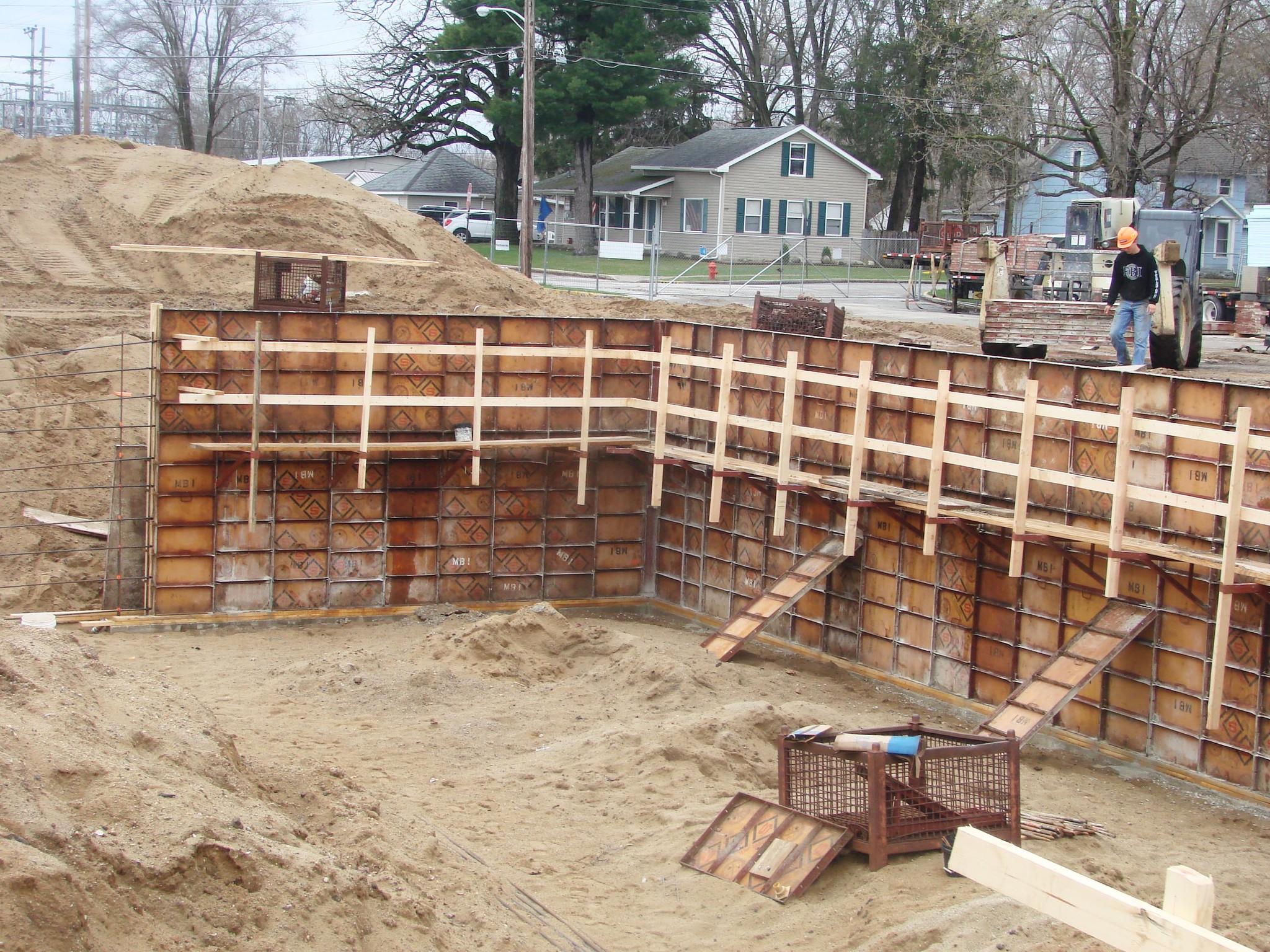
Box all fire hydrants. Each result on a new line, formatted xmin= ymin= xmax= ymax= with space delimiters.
xmin=707 ymin=262 xmax=718 ymax=280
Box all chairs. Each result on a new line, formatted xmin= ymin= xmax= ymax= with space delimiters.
xmin=544 ymin=231 xmax=555 ymax=244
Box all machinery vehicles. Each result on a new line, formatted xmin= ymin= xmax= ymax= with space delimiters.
xmin=976 ymin=192 xmax=1205 ymax=370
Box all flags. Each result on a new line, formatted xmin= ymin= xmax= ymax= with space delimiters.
xmin=591 ymin=202 xmax=598 ymax=216
xmin=536 ymin=195 xmax=553 ymax=234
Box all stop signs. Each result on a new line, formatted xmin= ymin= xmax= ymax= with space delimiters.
xmin=938 ymin=224 xmax=964 ymax=247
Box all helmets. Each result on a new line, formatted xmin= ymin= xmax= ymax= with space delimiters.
xmin=1117 ymin=227 xmax=1138 ymax=248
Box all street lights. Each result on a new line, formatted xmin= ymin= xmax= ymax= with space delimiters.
xmin=475 ymin=7 xmax=534 ymax=276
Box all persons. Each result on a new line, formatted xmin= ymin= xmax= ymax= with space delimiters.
xmin=1104 ymin=226 xmax=1160 ymax=369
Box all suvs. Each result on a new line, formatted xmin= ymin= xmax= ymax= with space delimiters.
xmin=444 ymin=209 xmax=544 ymax=244
xmin=416 ymin=205 xmax=460 ymax=224
